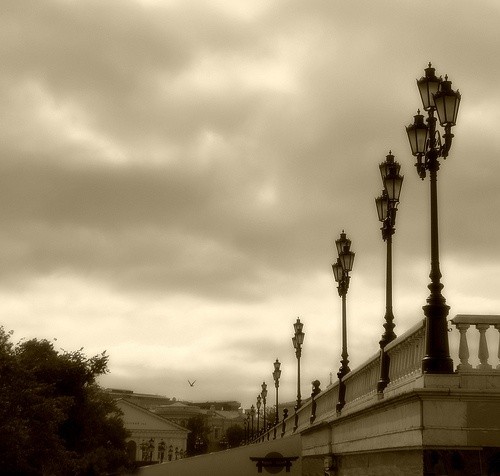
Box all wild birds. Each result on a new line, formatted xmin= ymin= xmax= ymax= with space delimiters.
xmin=187 ymin=377 xmax=199 ymax=389
